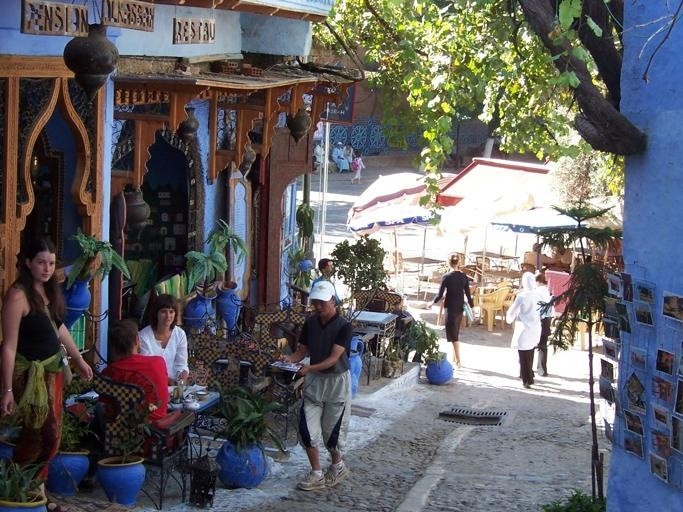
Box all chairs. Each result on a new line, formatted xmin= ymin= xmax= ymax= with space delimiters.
xmin=457 ymin=253 xmax=476 ymax=275
xmin=496 ymin=280 xmax=508 ymax=289
xmin=478 ymin=287 xmax=512 ymax=332
xmin=92 ymin=369 xmax=196 ymax=510
xmin=269 ymin=322 xmax=299 ymax=353
xmin=476 ymin=257 xmax=496 ymax=284
xmin=577 ymin=253 xmax=624 ymax=273
xmin=368 ymin=299 xmax=386 ymax=313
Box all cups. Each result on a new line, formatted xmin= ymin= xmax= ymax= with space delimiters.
xmin=172 ymin=388 xmax=182 ymax=404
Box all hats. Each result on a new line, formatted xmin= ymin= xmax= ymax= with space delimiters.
xmin=309 ymin=281 xmax=336 ymax=301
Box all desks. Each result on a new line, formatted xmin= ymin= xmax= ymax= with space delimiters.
xmin=400 ymin=257 xmax=446 ymax=301
xmin=489 ymin=270 xmax=522 ymax=289
xmin=470 ymin=251 xmax=520 ymax=270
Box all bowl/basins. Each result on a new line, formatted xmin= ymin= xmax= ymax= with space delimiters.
xmin=196 ymin=392 xmax=209 ymax=400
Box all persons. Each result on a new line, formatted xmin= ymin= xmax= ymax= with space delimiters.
xmin=314 ymin=141 xmax=333 ymax=174
xmin=532 ymin=275 xmax=554 ymax=376
xmin=505 ymin=271 xmax=542 ymax=389
xmin=332 ymin=142 xmax=349 ymax=173
xmin=428 ymin=253 xmax=475 ymax=368
xmin=351 ymin=152 xmax=365 ymax=184
xmin=392 ymin=299 xmax=430 ymax=363
xmin=277 ymin=281 xmax=354 ymax=491
xmin=311 ymin=258 xmax=341 ymax=307
xmin=2 ymin=235 xmax=93 ymax=511
xmin=343 ymin=142 xmax=356 ymax=172
xmin=100 ymin=318 xmax=168 ymax=423
xmin=525 ymin=242 xmax=555 ymax=271
xmin=138 ymin=294 xmax=189 ymax=386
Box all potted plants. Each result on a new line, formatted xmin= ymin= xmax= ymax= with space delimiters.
xmin=204 ymin=219 xmax=248 ymax=340
xmin=57 ymin=227 xmax=131 ymax=331
xmin=0 ymin=419 xmax=23 ymax=460
xmin=382 ymin=340 xmax=405 ymax=378
xmin=407 ymin=320 xmax=454 ymax=385
xmin=214 ymin=380 xmax=283 ymax=489
xmin=97 ymin=400 xmax=163 ymax=509
xmin=183 ymin=251 xmax=228 ymax=338
xmin=0 ymin=456 xmax=47 ymax=512
xmin=43 ymin=409 xmax=89 ymax=496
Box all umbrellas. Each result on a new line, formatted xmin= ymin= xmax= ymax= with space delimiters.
xmin=348 ymin=173 xmax=428 ymax=225
xmin=350 ymin=204 xmax=441 ymax=277
xmin=491 ymin=201 xmax=623 ymax=267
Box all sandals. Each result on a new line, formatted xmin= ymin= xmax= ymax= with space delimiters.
xmin=46 ymin=497 xmax=68 ymax=512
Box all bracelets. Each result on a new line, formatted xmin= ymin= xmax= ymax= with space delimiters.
xmin=3 ymin=388 xmax=13 ymax=392
xmin=72 ymin=354 xmax=83 ymax=361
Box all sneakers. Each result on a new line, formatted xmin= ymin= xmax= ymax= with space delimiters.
xmin=325 ymin=462 xmax=349 ymax=487
xmin=297 ymin=471 xmax=325 ymax=489
